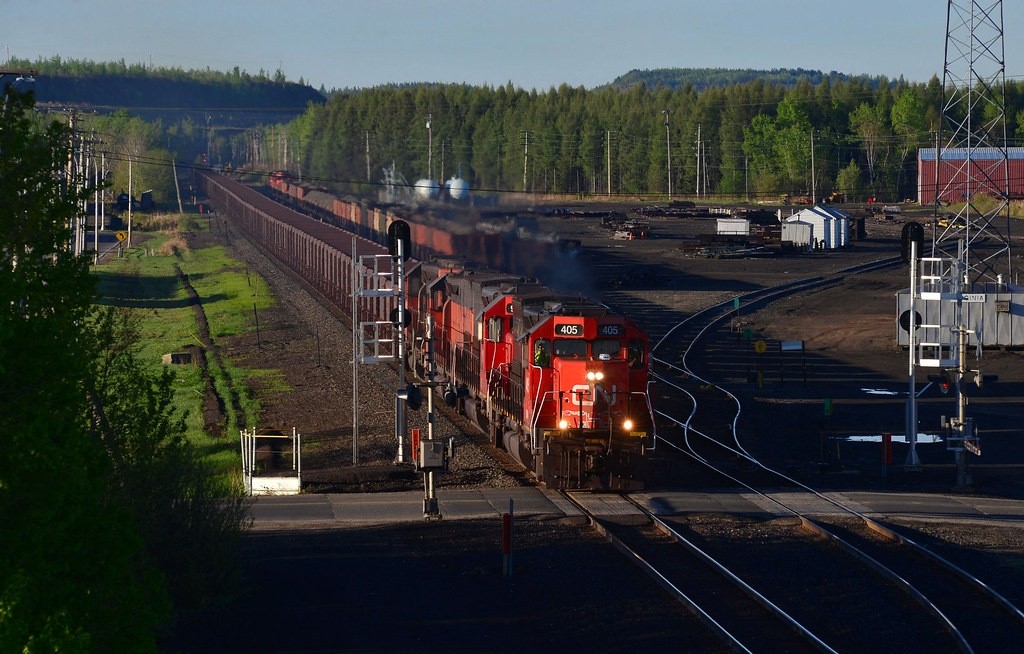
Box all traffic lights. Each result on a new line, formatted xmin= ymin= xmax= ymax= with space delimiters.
xmin=443 ymin=391 xmax=457 ymax=408
xmin=407 ymin=388 xmax=422 ymax=411
xmin=973 ymin=369 xmax=1000 ymax=389
xmin=927 ymin=370 xmax=951 ymax=393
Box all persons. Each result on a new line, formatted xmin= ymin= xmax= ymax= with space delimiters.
xmin=535 ymin=344 xmax=549 ymax=365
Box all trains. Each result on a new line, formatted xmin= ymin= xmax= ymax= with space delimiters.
xmin=190 ymin=164 xmax=657 ymax=493
xmin=269 ymin=170 xmax=583 ymax=285
